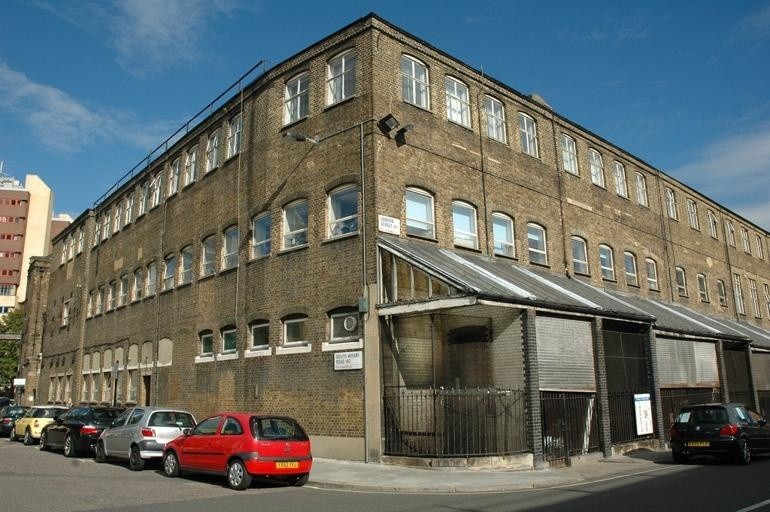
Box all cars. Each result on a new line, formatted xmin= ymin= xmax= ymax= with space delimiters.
xmin=670 ymin=402 xmax=770 ymax=465
